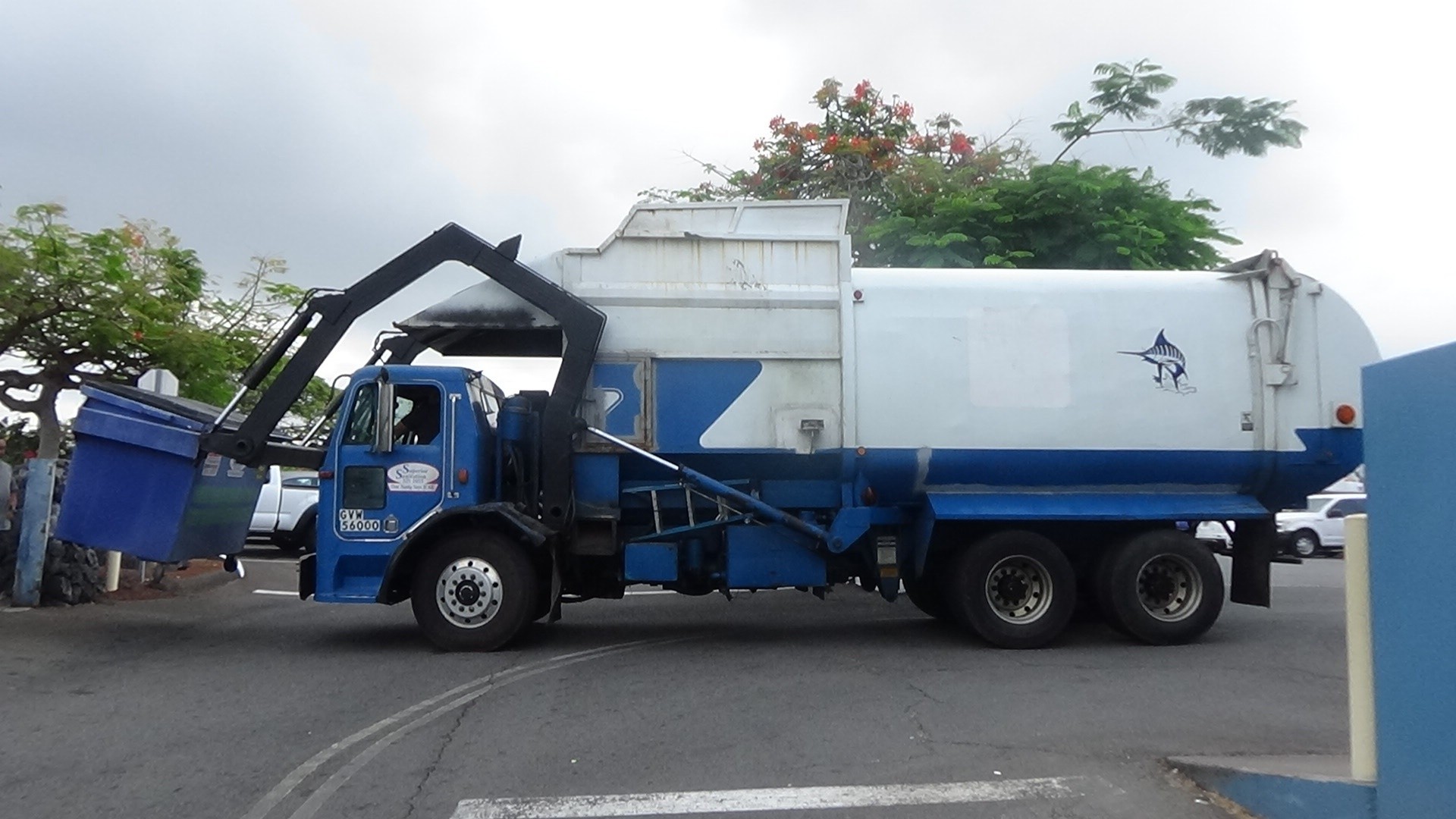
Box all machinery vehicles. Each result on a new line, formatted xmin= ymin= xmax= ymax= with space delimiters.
xmin=48 ymin=192 xmax=1387 ymax=650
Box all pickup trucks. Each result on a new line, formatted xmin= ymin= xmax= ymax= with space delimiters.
xmin=244 ymin=463 xmax=323 ymax=551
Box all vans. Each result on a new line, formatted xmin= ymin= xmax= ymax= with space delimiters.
xmin=1192 ymin=491 xmax=1369 ymax=558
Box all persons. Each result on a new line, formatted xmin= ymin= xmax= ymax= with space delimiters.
xmin=394 ymin=385 xmax=440 ymax=436
